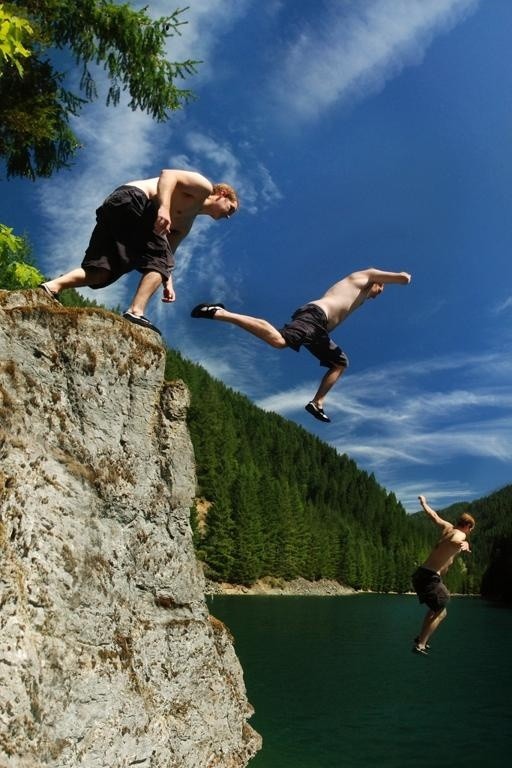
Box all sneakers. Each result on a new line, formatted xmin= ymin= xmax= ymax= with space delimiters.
xmin=411 ymin=637 xmax=433 ymax=659
xmin=188 ymin=302 xmax=226 ymax=320
xmin=305 ymin=400 xmax=333 ymax=424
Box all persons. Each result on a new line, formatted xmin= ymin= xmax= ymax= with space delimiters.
xmin=190 ymin=267 xmax=410 ymax=424
xmin=39 ymin=169 xmax=238 ymax=337
xmin=412 ymin=496 xmax=476 ymax=656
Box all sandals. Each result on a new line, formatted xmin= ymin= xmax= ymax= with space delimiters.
xmin=122 ymin=311 xmax=165 ymax=338
xmin=36 ymin=281 xmax=65 ymax=308
